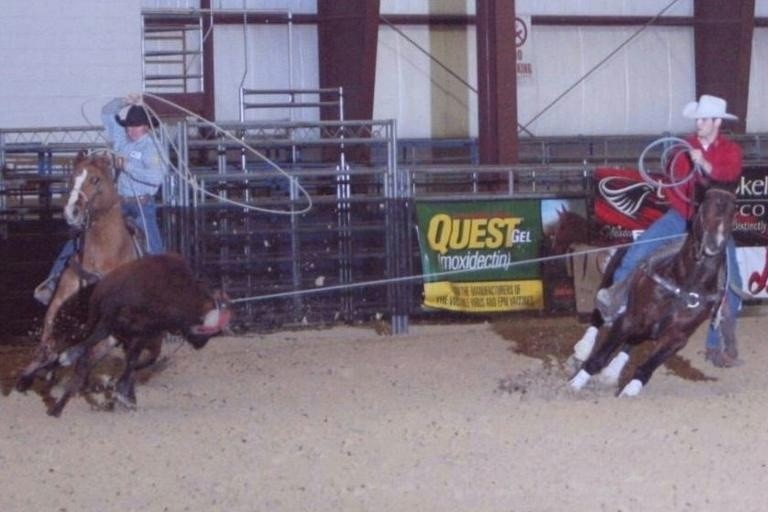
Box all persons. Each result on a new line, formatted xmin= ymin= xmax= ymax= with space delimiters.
xmin=596 ymin=95 xmax=743 ymax=368
xmin=33 ymin=92 xmax=167 ymax=306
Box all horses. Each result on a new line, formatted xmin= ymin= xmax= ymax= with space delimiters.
xmin=38 ymin=148 xmax=144 ymax=391
xmin=566 ymin=176 xmax=740 ymax=400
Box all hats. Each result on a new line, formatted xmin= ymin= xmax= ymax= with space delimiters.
xmin=683 ymin=94 xmax=739 ymax=120
xmin=114 ymin=105 xmax=160 ymax=127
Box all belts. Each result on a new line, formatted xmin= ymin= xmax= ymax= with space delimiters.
xmin=123 ymin=195 xmax=151 ymax=204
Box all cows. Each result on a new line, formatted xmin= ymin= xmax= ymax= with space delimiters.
xmin=15 ymin=251 xmax=239 ymax=413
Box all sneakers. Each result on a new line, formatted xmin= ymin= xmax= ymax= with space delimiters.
xmin=33 ymin=286 xmax=54 ymax=306
xmin=706 ymin=351 xmax=740 ymax=366
